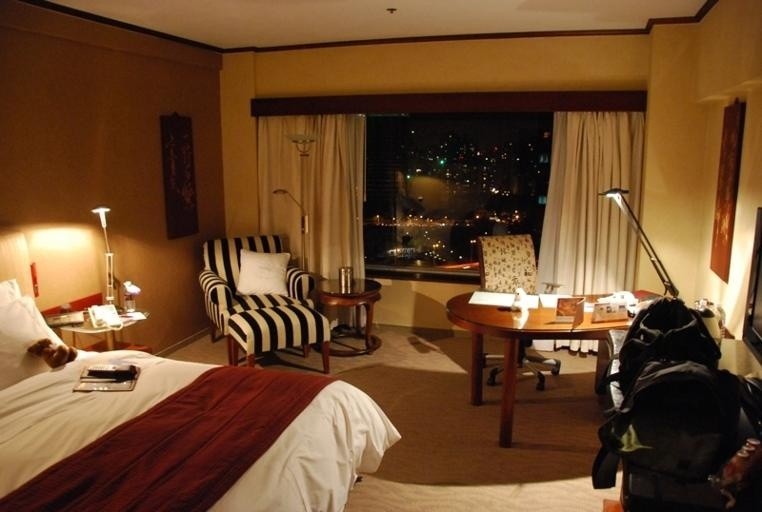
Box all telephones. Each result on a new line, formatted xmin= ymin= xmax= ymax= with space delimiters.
xmin=88 ymin=304 xmax=124 ymax=331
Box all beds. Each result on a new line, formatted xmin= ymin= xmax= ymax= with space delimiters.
xmin=0 ymin=228 xmax=400 ymax=512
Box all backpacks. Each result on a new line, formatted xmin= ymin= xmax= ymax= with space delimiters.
xmin=590 ymin=296 xmax=761 ymax=512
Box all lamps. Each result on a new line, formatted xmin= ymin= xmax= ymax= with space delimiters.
xmin=274 ymin=135 xmax=318 ymax=270
xmin=91 ymin=205 xmax=123 ymax=305
xmin=598 ymin=188 xmax=681 ymax=301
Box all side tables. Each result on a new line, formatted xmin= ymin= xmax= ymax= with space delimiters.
xmin=314 ymin=279 xmax=381 ymax=357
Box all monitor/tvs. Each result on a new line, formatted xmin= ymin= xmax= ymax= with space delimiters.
xmin=749 ymin=249 xmax=762 ymax=359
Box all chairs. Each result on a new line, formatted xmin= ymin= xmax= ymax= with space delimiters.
xmin=478 ymin=234 xmax=561 ymax=390
xmin=199 ymin=235 xmax=314 ymax=363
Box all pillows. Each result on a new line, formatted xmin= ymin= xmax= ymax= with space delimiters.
xmin=0 ymin=297 xmax=66 ymax=387
xmin=0 ymin=279 xmax=21 ymax=305
xmin=235 ymin=248 xmax=291 ymax=296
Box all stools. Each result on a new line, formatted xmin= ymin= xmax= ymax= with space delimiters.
xmin=229 ymin=304 xmax=331 ymax=373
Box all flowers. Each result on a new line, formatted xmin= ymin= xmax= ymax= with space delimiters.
xmin=124 ymin=279 xmax=140 ymax=300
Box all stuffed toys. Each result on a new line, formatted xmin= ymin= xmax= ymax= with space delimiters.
xmin=28 ymin=338 xmax=79 ymax=367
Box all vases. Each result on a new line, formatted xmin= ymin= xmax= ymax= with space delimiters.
xmin=124 ymin=300 xmax=136 ymax=312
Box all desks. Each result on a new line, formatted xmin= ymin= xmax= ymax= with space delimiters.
xmin=605 ymin=330 xmax=762 ymax=512
xmin=56 ymin=309 xmax=152 ymax=353
xmin=447 ymin=291 xmax=662 ymax=448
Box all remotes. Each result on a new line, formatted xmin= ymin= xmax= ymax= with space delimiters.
xmin=87 ymin=363 xmax=132 ymax=375
